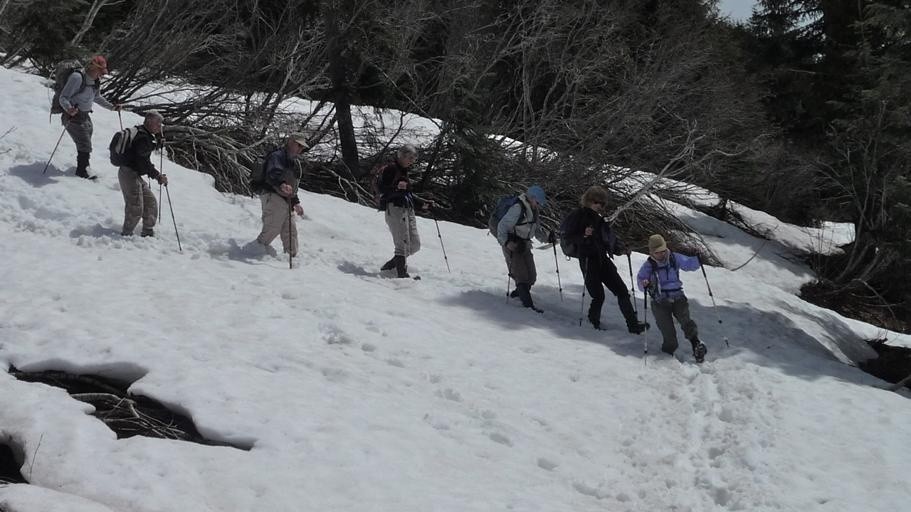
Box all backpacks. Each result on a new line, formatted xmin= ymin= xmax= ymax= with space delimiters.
xmin=107 ymin=123 xmax=147 ymax=167
xmin=560 ymin=211 xmax=584 ymax=258
xmin=249 ymin=162 xmax=268 ymax=195
xmin=372 ymin=163 xmax=389 ymax=198
xmin=488 ymin=191 xmax=527 ymax=239
xmin=49 ymin=58 xmax=87 ymax=114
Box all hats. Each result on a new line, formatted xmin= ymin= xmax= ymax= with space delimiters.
xmin=527 ymin=185 xmax=549 ymax=204
xmin=289 ymin=131 xmax=311 ymax=148
xmin=647 ymin=234 xmax=668 ymax=256
xmin=581 ymin=186 xmax=609 ymax=209
xmin=93 ymin=55 xmax=110 ymax=74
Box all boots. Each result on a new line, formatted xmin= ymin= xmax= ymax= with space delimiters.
xmin=380 ymin=253 xmax=396 ymax=272
xmin=585 ymin=299 xmax=607 ymax=333
xmin=395 ymin=253 xmax=420 ymax=282
xmin=75 ymin=151 xmax=97 ymax=180
xmin=618 ymin=297 xmax=650 ymax=334
xmin=510 ymin=284 xmax=532 ymax=299
xmin=689 ymin=336 xmax=707 ymax=365
xmin=517 ymin=283 xmax=544 ymax=314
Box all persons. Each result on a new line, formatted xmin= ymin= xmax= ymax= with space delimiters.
xmin=379 ymin=144 xmax=422 ymax=281
xmin=636 ymin=234 xmax=708 ymax=362
xmin=495 ymin=186 xmax=549 ymax=314
xmin=560 ymin=185 xmax=651 ymax=335
xmin=251 ymin=130 xmax=310 ymax=257
xmin=118 ymin=109 xmax=168 ymax=236
xmin=58 ymin=55 xmax=122 ymax=179
